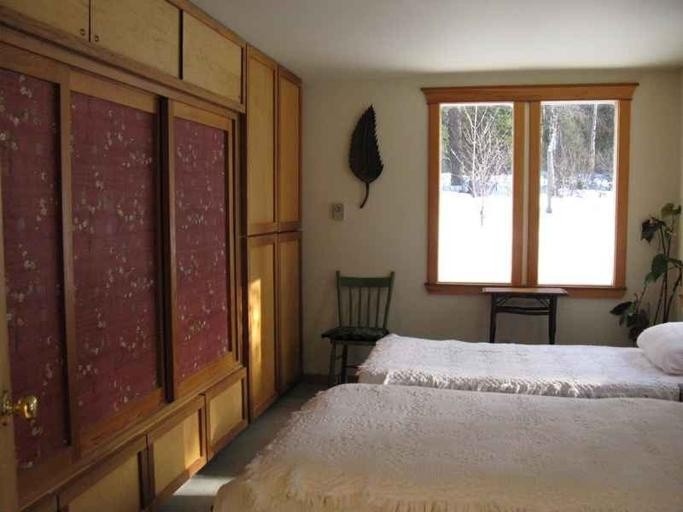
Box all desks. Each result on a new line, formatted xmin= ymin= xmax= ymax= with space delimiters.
xmin=479 ymin=285 xmax=572 ymax=345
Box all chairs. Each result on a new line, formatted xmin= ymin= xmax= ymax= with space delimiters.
xmin=321 ymin=270 xmax=396 ymax=384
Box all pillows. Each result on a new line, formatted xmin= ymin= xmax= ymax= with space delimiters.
xmin=635 ymin=318 xmax=683 ymax=377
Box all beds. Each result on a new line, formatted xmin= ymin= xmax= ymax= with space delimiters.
xmin=354 ymin=332 xmax=682 ymax=401
xmin=207 ymin=381 xmax=682 ymax=512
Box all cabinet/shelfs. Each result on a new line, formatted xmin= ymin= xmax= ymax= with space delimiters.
xmin=245 ymin=50 xmax=276 ymax=421
xmin=149 ymin=367 xmax=248 ymax=502
xmin=181 ymin=11 xmax=242 ymax=105
xmin=275 ymin=60 xmax=302 ymax=402
xmin=3 ymin=0 xmax=179 ymax=79
xmin=0 ymin=43 xmax=243 ymax=511
xmin=56 ymin=429 xmax=151 ymax=511
